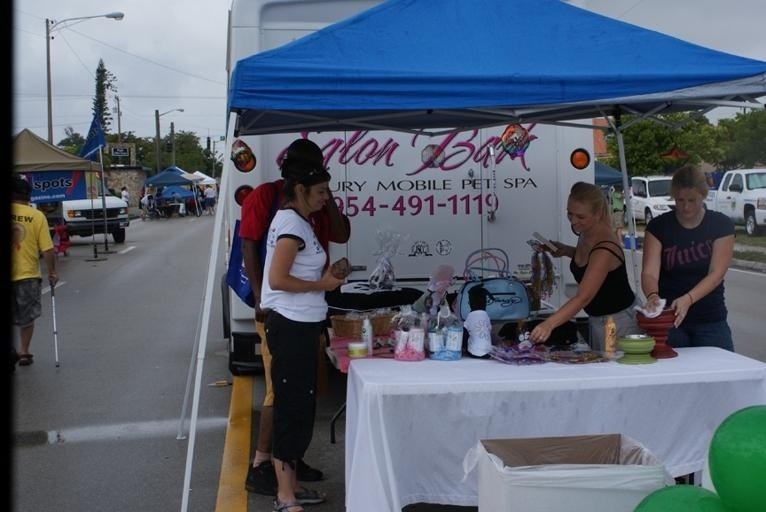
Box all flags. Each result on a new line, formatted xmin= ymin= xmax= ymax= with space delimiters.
xmin=79 ymin=110 xmax=106 ymax=160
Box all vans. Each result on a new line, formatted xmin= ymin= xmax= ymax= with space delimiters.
xmin=59 ymin=178 xmax=130 ymax=243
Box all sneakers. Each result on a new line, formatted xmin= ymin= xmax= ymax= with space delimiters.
xmin=245 ymin=460 xmax=278 ymax=496
xmin=296 ymin=456 xmax=323 ymax=481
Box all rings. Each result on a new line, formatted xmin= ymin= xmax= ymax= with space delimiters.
xmin=538 ymin=334 xmax=544 ymax=343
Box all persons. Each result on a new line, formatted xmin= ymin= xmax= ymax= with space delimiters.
xmin=609 ymin=183 xmax=627 ymax=246
xmin=238 ymin=138 xmax=352 ymax=497
xmin=642 ymin=163 xmax=738 ymax=353
xmin=11 ymin=176 xmax=57 ymax=366
xmin=120 ymin=186 xmax=130 ymax=207
xmin=137 ymin=183 xmax=215 ymax=221
xmin=528 ymin=181 xmax=648 ymax=351
xmin=238 ymin=164 xmax=345 ymax=510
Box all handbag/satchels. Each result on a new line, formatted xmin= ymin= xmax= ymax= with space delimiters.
xmin=225 ymin=182 xmax=279 ymax=308
xmin=454 ymin=248 xmax=531 ymax=322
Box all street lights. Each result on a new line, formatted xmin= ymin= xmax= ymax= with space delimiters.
xmin=42 ymin=8 xmax=128 ymax=150
xmin=150 ymin=108 xmax=186 ymax=172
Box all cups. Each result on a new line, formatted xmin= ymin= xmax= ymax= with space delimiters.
xmin=348 ymin=342 xmax=367 ymax=358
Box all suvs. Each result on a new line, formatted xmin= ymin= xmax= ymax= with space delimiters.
xmin=622 ymin=177 xmax=676 ymax=224
xmin=714 ymin=168 xmax=766 ymax=235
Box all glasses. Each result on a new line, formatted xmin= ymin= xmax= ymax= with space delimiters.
xmin=295 ymin=165 xmax=326 ymax=185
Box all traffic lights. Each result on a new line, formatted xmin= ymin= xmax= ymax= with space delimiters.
xmin=206 ymin=137 xmax=211 ymax=158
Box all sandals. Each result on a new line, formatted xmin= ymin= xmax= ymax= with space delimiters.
xmin=18 ymin=354 xmax=33 ymax=365
xmin=272 ymin=497 xmax=305 ymax=511
xmin=294 ymin=485 xmax=326 ymax=505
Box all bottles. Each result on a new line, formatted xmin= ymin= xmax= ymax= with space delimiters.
xmin=605 ymin=316 xmax=617 ymax=352
xmin=389 ymin=296 xmax=464 ymax=358
xmin=361 ymin=315 xmax=374 ymax=355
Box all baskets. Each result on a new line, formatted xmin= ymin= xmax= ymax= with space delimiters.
xmin=330 ymin=312 xmax=399 ymax=338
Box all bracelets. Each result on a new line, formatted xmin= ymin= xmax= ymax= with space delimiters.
xmin=645 ymin=291 xmax=658 ymax=299
xmin=687 ymin=290 xmax=694 ymax=304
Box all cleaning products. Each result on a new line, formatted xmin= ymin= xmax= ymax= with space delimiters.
xmin=605 ymin=315 xmax=618 ymax=356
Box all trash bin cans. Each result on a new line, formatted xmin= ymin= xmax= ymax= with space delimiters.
xmin=474 ymin=434 xmax=666 ymax=512
